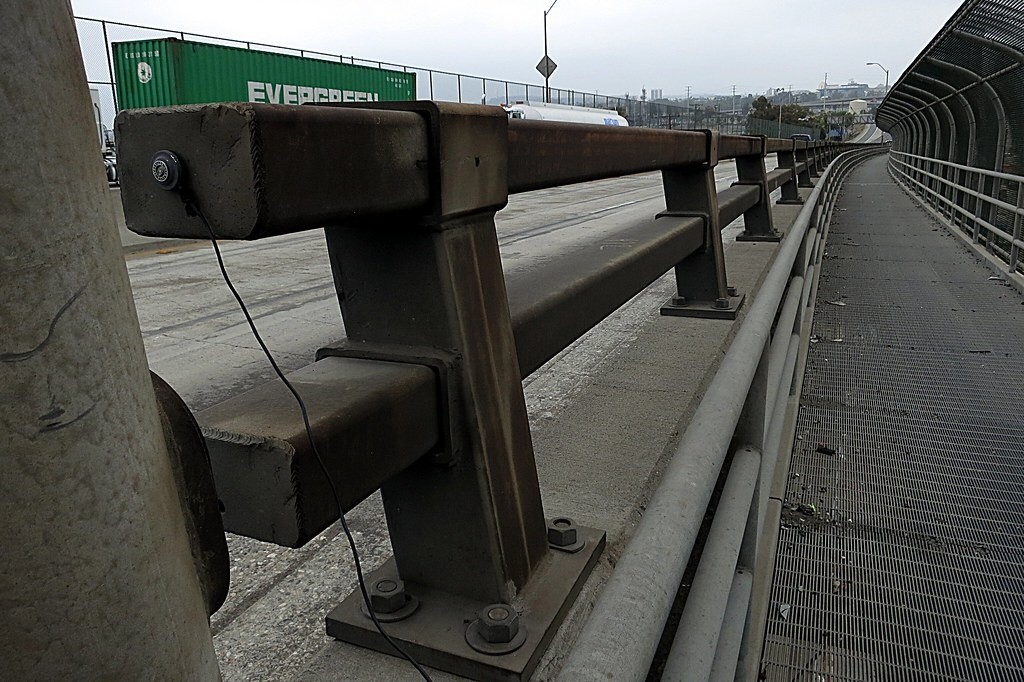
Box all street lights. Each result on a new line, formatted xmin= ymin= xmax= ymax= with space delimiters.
xmin=866 ymin=62 xmax=889 ymax=145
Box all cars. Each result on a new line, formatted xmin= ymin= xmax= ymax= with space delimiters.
xmin=790 ymin=134 xmax=813 ymax=141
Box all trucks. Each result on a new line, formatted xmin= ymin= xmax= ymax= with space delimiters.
xmin=500 ymin=100 xmax=630 ymax=128
